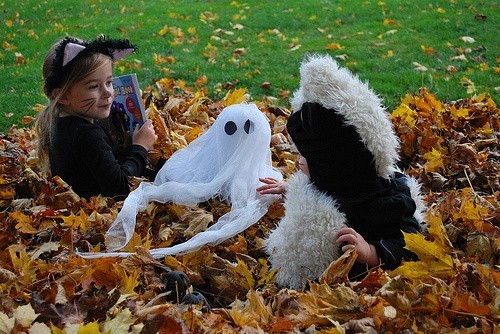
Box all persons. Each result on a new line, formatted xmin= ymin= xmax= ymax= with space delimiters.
xmin=161 ymin=51 xmax=429 ymax=315
xmin=34 ymin=33 xmax=169 ymax=203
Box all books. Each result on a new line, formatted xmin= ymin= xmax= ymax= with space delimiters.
xmin=94 ymin=72 xmax=148 ymax=151
